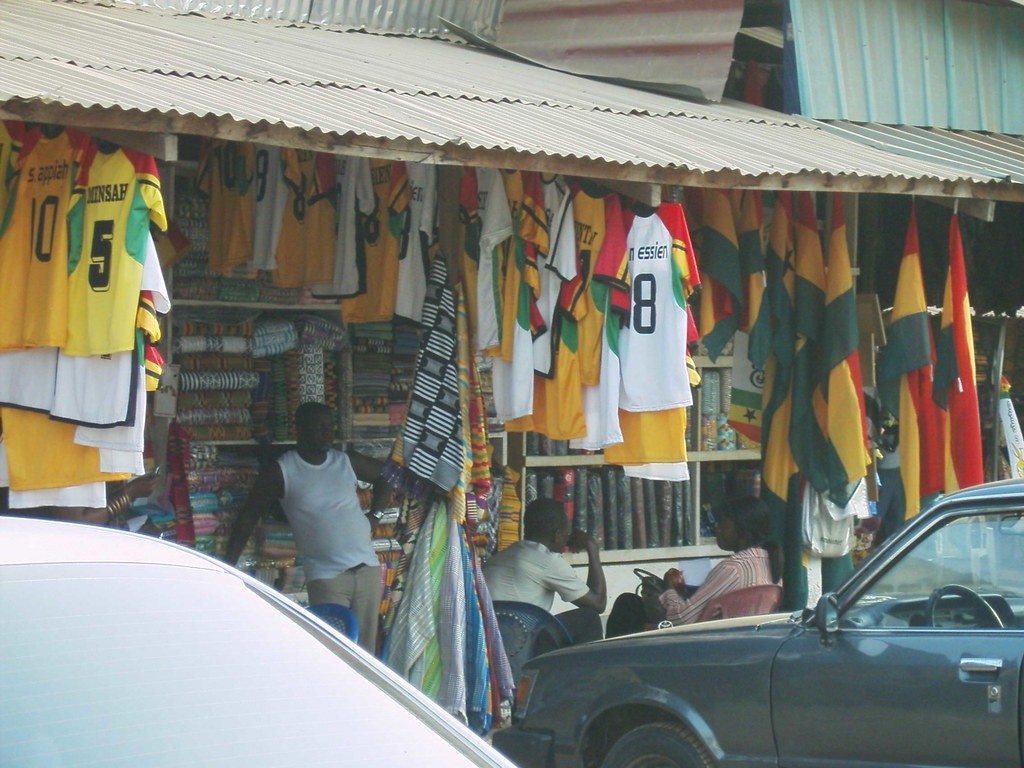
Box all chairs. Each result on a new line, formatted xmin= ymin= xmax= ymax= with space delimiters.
xmin=642 ymin=585 xmax=782 ymax=631
xmin=492 ymin=601 xmax=573 ymax=683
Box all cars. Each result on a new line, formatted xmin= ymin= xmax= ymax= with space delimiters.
xmin=0 ymin=516 xmax=517 ymax=768
xmin=492 ymin=476 xmax=1024 ymax=768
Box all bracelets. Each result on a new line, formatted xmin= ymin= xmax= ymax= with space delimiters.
xmin=369 ymin=508 xmax=385 ymax=520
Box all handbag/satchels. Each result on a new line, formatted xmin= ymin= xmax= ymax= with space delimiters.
xmin=803 ymin=481 xmax=854 ymax=558
xmin=633 ymin=568 xmax=699 ymax=625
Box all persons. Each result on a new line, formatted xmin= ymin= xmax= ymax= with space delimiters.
xmin=605 ymin=495 xmax=783 ymax=639
xmin=224 ymin=402 xmax=395 ymax=658
xmin=482 ymin=498 xmax=607 ymax=644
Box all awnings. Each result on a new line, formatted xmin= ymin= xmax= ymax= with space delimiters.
xmin=0 ymin=3 xmax=1024 ymax=203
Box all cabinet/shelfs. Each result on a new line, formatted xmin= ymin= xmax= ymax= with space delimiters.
xmin=169 ymin=300 xmax=800 ymax=638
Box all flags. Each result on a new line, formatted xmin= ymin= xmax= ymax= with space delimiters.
xmin=681 ymin=186 xmax=983 ymax=611
